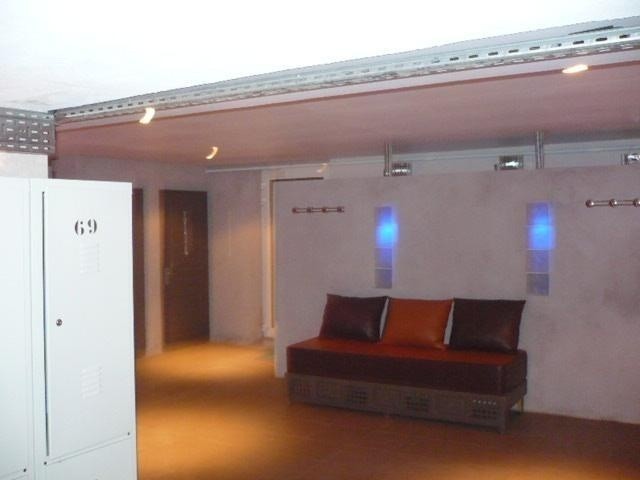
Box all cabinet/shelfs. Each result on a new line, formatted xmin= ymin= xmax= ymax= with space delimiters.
xmin=1 ymin=178 xmax=137 ymax=480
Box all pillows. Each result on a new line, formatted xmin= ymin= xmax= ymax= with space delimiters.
xmin=319 ymin=294 xmax=388 ymax=341
xmin=381 ymin=298 xmax=452 ymax=350
xmin=450 ymin=298 xmax=526 ymax=353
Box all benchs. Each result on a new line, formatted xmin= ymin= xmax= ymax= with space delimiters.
xmin=284 ymin=336 xmax=527 ymax=435
